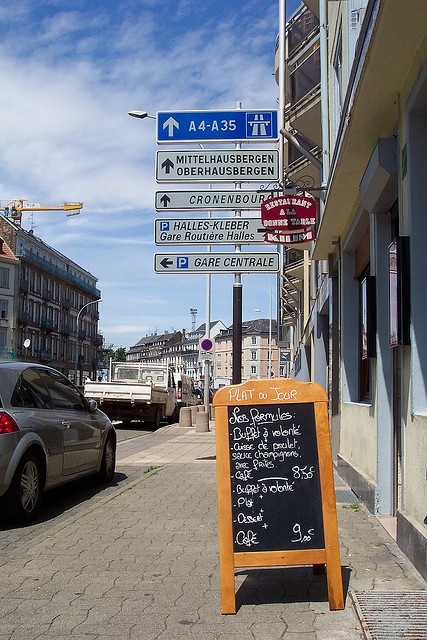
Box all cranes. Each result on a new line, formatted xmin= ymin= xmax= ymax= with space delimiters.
xmin=0 ymin=198 xmax=82 ymax=226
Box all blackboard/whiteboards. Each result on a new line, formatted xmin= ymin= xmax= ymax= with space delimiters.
xmin=212 ymin=378 xmax=341 ymax=568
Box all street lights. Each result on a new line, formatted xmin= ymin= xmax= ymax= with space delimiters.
xmin=127 ymin=111 xmax=213 ymax=409
xmin=254 ymin=308 xmax=269 ymax=317
xmin=73 ymin=298 xmax=102 ymax=384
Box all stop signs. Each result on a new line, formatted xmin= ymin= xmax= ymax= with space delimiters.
xmin=200 ymin=339 xmax=213 ymax=352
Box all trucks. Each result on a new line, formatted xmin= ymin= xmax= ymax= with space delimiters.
xmin=84 ymin=358 xmax=178 ymax=427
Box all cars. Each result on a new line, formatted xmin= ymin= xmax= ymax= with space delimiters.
xmin=0 ymin=363 xmax=116 ymax=517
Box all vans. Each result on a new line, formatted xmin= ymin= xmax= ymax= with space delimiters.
xmin=172 ymin=373 xmax=196 ymax=412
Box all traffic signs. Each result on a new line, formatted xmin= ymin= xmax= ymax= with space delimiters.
xmin=154 ymin=108 xmax=280 ymax=143
xmin=153 ymin=252 xmax=279 ymax=274
xmin=153 ymin=217 xmax=275 ymax=246
xmin=153 ymin=190 xmax=272 ymax=211
xmin=154 ymin=149 xmax=280 ymax=182
